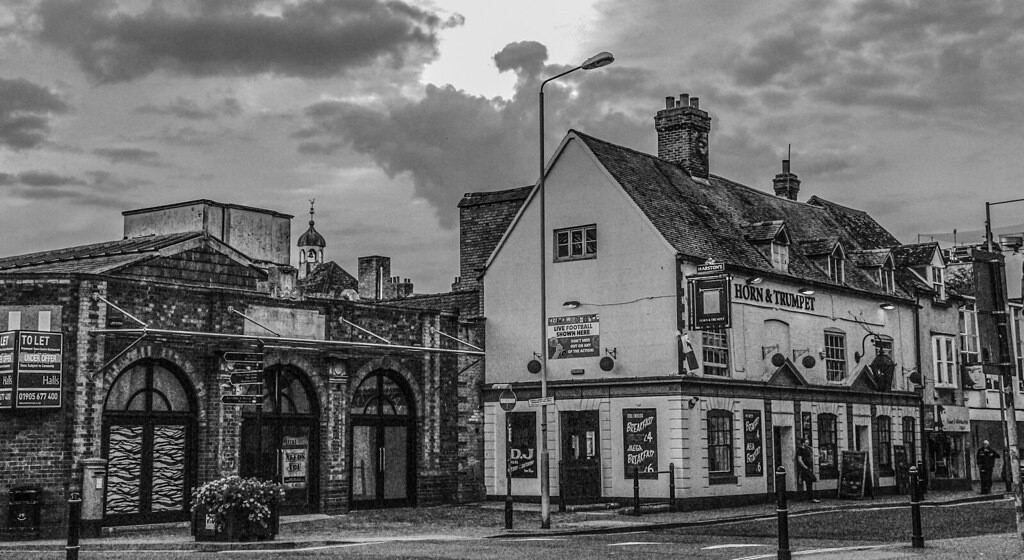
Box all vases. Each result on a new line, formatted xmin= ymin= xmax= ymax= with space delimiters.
xmin=191 ymin=501 xmax=279 ymax=542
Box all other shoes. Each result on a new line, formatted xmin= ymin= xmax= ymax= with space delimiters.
xmin=809 ymin=499 xmax=820 ymax=503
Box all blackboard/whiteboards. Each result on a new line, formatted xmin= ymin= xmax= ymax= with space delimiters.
xmin=839 ymin=450 xmax=872 ymax=497
xmin=892 ymin=445 xmax=909 ymax=483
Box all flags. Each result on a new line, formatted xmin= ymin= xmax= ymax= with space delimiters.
xmin=681 ymin=334 xmax=699 ymax=371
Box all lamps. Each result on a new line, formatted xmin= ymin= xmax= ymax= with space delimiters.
xmin=879 ymin=302 xmax=895 ymax=310
xmin=798 ymin=288 xmax=816 ymax=295
xmin=793 ymin=347 xmax=815 ymax=368
xmin=600 ymin=347 xmax=618 ymax=372
xmin=762 ymin=344 xmax=783 ymax=366
xmin=562 ymin=300 xmax=581 ymax=310
xmin=906 ymin=372 xmax=920 ymax=389
xmin=855 ymin=333 xmax=894 ymax=392
xmin=748 ymin=275 xmax=762 ymax=284
xmin=527 ymin=351 xmax=542 ymax=374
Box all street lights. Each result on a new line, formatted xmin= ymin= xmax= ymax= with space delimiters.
xmin=536 ymin=51 xmax=615 ymax=531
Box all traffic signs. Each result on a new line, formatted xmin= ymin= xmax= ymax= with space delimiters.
xmin=229 ymin=371 xmax=264 ymax=385
xmin=221 ymin=395 xmax=264 ymax=405
xmin=222 ymin=352 xmax=264 ymax=364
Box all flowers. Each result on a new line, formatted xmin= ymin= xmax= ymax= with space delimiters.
xmin=190 ymin=475 xmax=286 ymax=531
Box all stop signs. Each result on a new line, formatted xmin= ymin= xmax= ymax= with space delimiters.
xmin=498 ymin=390 xmax=517 ymax=411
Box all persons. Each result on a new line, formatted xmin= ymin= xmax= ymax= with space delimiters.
xmin=976 ymin=439 xmax=1000 ymax=495
xmin=964 ymin=365 xmax=986 ymax=389
xmin=797 ymin=438 xmax=821 ymax=503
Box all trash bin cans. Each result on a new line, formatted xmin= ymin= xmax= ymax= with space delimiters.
xmin=8 ymin=486 xmax=41 ymax=541
